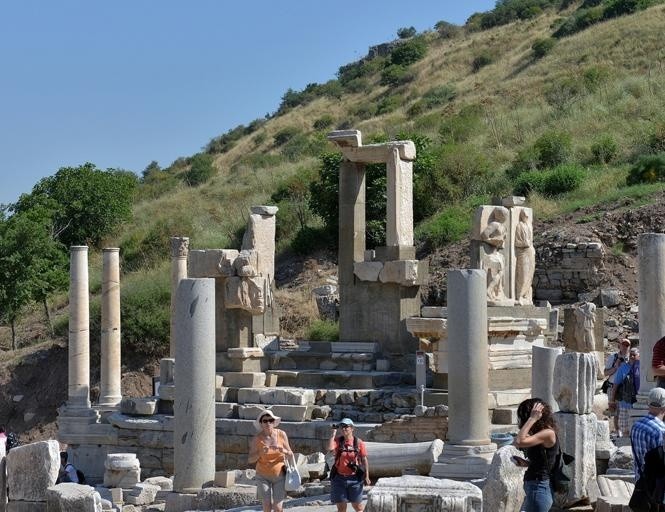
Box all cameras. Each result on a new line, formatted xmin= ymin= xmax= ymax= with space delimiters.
xmin=332 ymin=423 xmax=339 ymax=429
xmin=348 ymin=461 xmax=358 ymax=474
xmin=619 ymin=357 xmax=628 ymax=362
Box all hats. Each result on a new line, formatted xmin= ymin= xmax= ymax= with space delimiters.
xmin=253 ymin=410 xmax=281 ymax=430
xmin=339 ymin=418 xmax=354 ymax=426
xmin=647 ymin=386 xmax=665 ymax=409
xmin=620 ymin=338 xmax=630 ymax=345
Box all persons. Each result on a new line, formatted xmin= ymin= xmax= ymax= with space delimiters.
xmin=0 ymin=424 xmax=13 ymax=457
xmin=507 ymin=398 xmax=561 ymax=512
xmin=248 ymin=410 xmax=294 ymax=512
xmin=604 ymin=338 xmax=665 ymax=512
xmin=57 ymin=451 xmax=84 ymax=484
xmin=326 ymin=417 xmax=371 ymax=512
xmin=516 ymin=207 xmax=538 ymax=302
xmin=482 ymin=208 xmax=508 ymax=300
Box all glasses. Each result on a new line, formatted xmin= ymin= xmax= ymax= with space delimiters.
xmin=263 ymin=419 xmax=274 ymax=423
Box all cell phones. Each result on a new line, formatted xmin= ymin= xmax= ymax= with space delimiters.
xmin=509 ymin=457 xmax=518 ymax=463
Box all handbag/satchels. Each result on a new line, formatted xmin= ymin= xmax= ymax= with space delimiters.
xmin=551 ymin=453 xmax=570 ymax=496
xmin=600 ymin=380 xmax=608 ymax=394
xmin=629 ymin=478 xmax=659 ymax=512
xmin=283 ymin=452 xmax=300 ymax=492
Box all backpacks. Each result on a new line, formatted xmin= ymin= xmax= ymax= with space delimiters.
xmin=65 ymin=464 xmax=85 ymax=484
